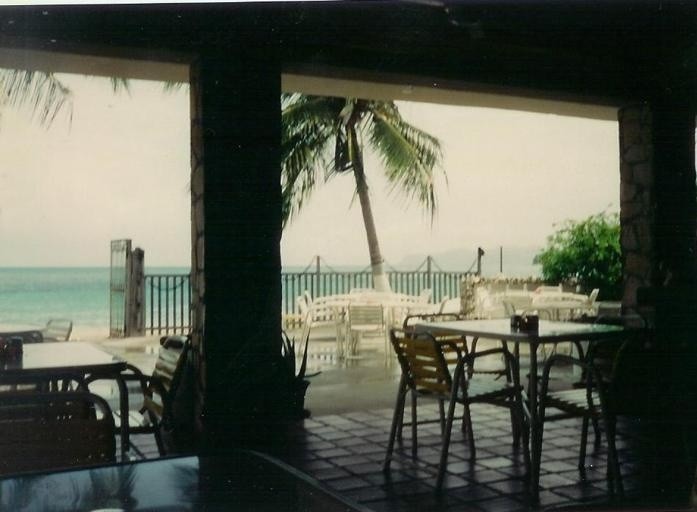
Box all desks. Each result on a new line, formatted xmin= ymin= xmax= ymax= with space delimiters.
xmin=0 ymin=449 xmax=378 ymax=512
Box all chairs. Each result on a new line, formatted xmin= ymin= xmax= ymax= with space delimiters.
xmin=383 ymin=328 xmax=523 ymax=487
xmin=344 ymin=303 xmax=390 ymax=370
xmin=572 ymin=305 xmax=646 ymax=445
xmin=0 ymin=318 xmax=193 ymax=474
xmin=297 ymin=296 xmax=347 ymax=362
xmin=533 ymin=330 xmax=694 ymax=490
xmin=297 ymin=282 xmax=695 ymax=329
xmin=403 ymin=311 xmax=473 ymax=450
